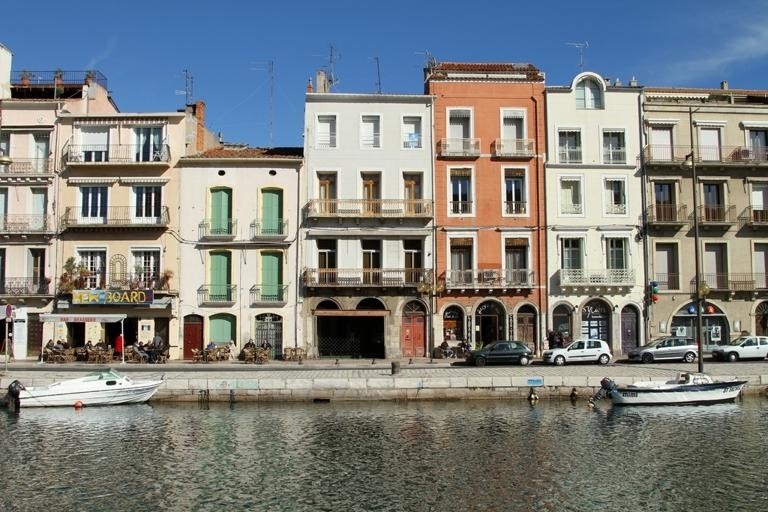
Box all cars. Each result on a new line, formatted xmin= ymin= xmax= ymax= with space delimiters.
xmin=628 ymin=336 xmax=699 ymax=363
xmin=464 ymin=341 xmax=533 ymax=366
xmin=542 ymin=339 xmax=613 ymax=365
xmin=712 ymin=336 xmax=768 ymax=362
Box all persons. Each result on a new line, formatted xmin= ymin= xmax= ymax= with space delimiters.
xmin=459 ymin=340 xmax=471 ymax=355
xmin=441 ymin=338 xmax=452 ymax=359
xmin=545 ymin=329 xmax=566 ymax=351
xmin=38 ymin=334 xmax=271 ymax=361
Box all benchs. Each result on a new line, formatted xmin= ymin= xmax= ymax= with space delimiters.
xmin=438 ymin=346 xmax=462 ymax=358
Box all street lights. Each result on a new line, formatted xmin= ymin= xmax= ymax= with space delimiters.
xmin=681 ymin=106 xmax=710 ymax=373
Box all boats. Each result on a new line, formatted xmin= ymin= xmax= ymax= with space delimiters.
xmin=4 ymin=367 xmax=165 ymax=407
xmin=587 ymin=372 xmax=749 ymax=408
xmin=594 ymin=401 xmax=742 ymax=418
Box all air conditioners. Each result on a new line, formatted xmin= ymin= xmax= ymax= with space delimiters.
xmin=483 ymin=268 xmax=498 ymax=279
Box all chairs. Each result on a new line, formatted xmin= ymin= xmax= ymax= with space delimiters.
xmin=40 ymin=343 xmax=306 ymax=364
